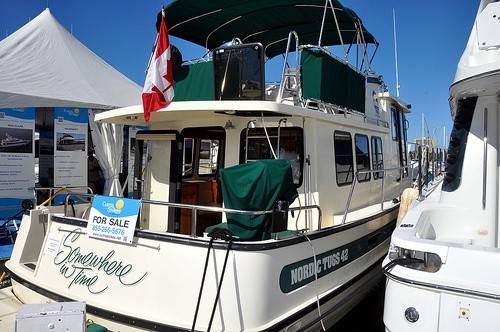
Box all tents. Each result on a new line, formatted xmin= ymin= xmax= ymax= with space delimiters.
xmin=0 ymin=8 xmax=143 ymax=109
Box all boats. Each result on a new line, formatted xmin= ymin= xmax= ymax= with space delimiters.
xmin=57 ymin=137 xmax=85 ymax=145
xmin=4 ymin=0 xmax=412 ymax=332
xmin=0 ymin=133 xmax=31 ymax=147
xmin=381 ymin=0 xmax=500 ymax=332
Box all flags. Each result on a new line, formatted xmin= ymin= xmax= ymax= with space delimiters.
xmin=142 ymin=7 xmax=174 ymax=123
